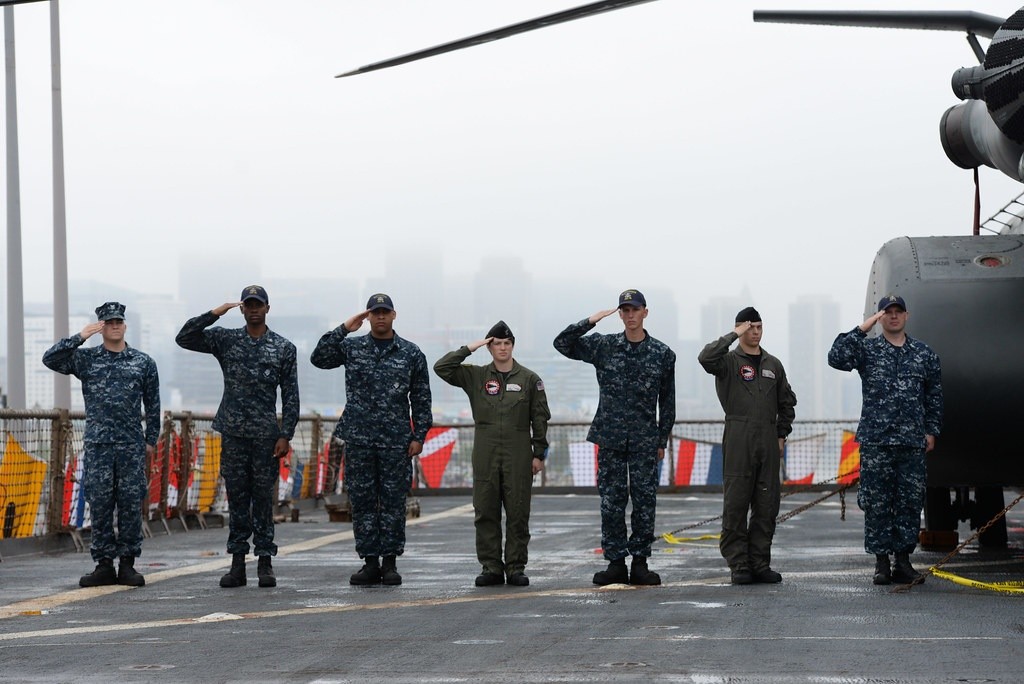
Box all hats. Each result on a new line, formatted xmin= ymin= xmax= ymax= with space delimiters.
xmin=877 ymin=295 xmax=907 ymax=312
xmin=617 ymin=289 xmax=647 ymax=309
xmin=489 ymin=320 xmax=513 ymax=339
xmin=241 ymin=286 xmax=269 ymax=304
xmin=366 ymin=294 xmax=394 ymax=312
xmin=735 ymin=306 xmax=762 ymax=322
xmin=95 ymin=302 xmax=126 ymax=321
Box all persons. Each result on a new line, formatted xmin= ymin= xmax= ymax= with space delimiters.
xmin=698 ymin=307 xmax=797 ymax=585
xmin=827 ymin=295 xmax=945 ymax=585
xmin=552 ymin=288 xmax=677 ymax=587
xmin=310 ymin=293 xmax=432 ymax=584
xmin=42 ymin=302 xmax=160 ymax=588
xmin=433 ymin=319 xmax=551 ymax=587
xmin=175 ymin=285 xmax=299 ymax=588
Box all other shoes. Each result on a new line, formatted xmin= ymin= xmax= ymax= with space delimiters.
xmin=756 ymin=568 xmax=783 ymax=583
xmin=507 ymin=573 xmax=529 ymax=586
xmin=475 ymin=573 xmax=505 ymax=586
xmin=730 ymin=569 xmax=754 ymax=584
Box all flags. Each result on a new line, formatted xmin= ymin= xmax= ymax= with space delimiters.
xmin=1 ymin=423 xmax=860 ymax=537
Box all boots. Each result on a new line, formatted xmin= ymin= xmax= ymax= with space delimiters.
xmin=630 ymin=555 xmax=661 ymax=585
xmin=593 ymin=559 xmax=630 ymax=585
xmin=349 ymin=557 xmax=381 ymax=585
xmin=256 ymin=556 xmax=280 ymax=587
xmin=118 ymin=560 xmax=145 ymax=586
xmin=381 ymin=556 xmax=402 ymax=585
xmin=873 ymin=554 xmax=893 ymax=585
xmin=892 ymin=552 xmax=925 ymax=584
xmin=220 ymin=553 xmax=247 ymax=587
xmin=79 ymin=558 xmax=117 ymax=587
